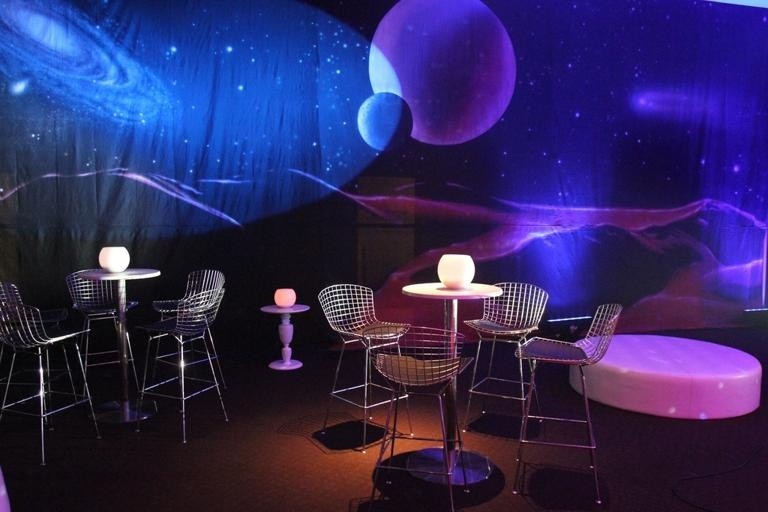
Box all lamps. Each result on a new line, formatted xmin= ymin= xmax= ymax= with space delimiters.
xmin=438 ymin=253 xmax=475 ymax=288
xmin=98 ymin=245 xmax=131 ymax=274
